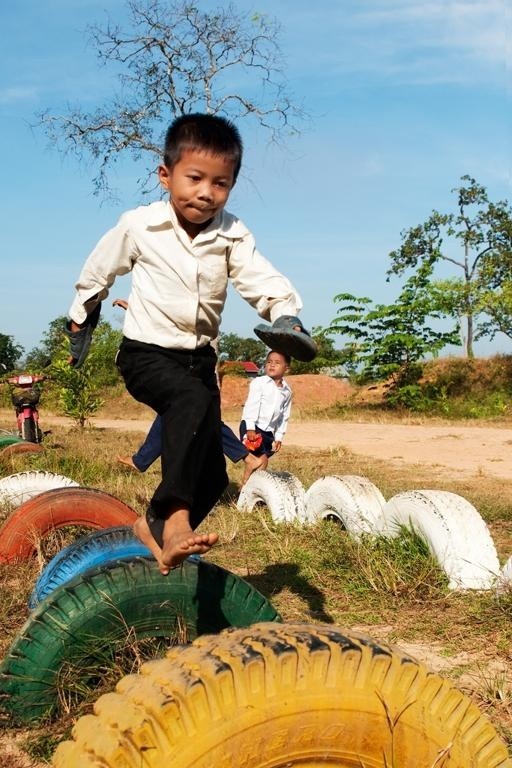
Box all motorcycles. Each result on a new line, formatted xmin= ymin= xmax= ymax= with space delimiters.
xmin=1 ymin=375 xmax=60 ymax=443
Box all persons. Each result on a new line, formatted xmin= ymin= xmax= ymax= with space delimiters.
xmin=235 ymin=347 xmax=299 ymax=491
xmin=59 ymin=113 xmax=315 ymax=577
xmin=111 ymin=293 xmax=263 ymax=492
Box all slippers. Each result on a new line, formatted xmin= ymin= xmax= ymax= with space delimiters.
xmin=65 ymin=301 xmax=101 ymax=369
xmin=253 ymin=315 xmax=317 ymax=362
